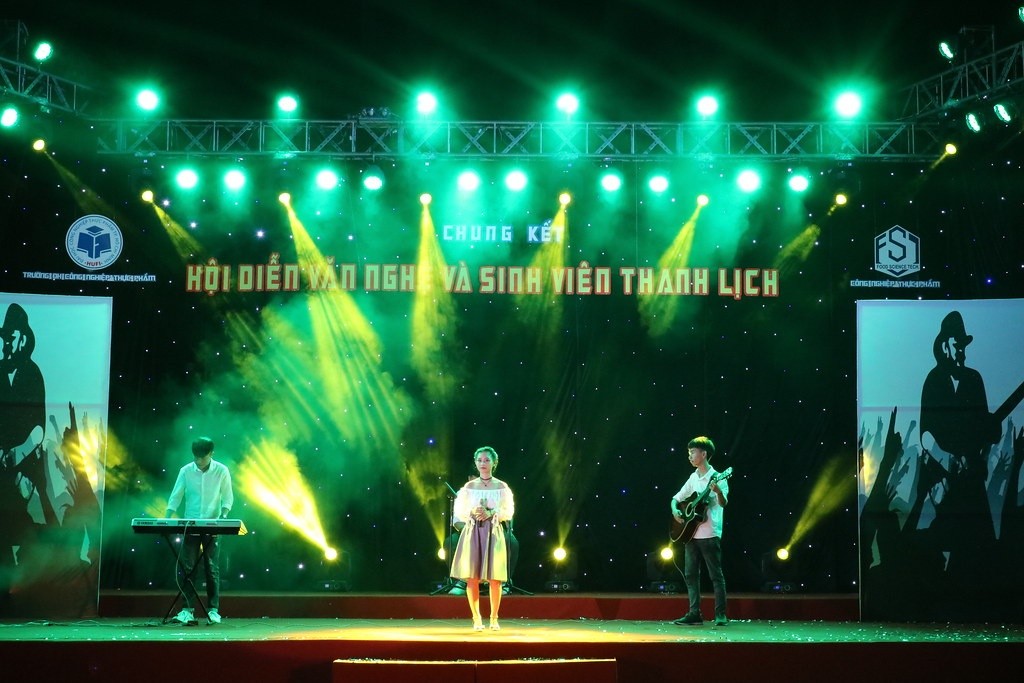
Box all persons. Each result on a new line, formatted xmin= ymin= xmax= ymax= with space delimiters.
xmin=443 ymin=446 xmax=519 ymax=632
xmin=671 ymin=436 xmax=729 ymax=626
xmin=165 ymin=437 xmax=233 ymax=625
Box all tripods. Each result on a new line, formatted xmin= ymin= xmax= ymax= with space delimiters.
xmin=426 ymin=493 xmax=535 ymax=597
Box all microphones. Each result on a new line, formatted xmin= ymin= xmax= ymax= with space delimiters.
xmin=445 ymin=481 xmax=457 ymax=499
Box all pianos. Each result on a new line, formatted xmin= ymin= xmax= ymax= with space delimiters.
xmin=130 ymin=517 xmax=248 ymax=536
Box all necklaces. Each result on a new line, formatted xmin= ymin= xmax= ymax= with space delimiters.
xmin=480 ymin=477 xmax=492 ymax=486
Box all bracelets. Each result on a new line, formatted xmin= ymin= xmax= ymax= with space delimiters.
xmin=719 ymin=492 xmax=722 ymax=495
xmin=221 ymin=514 xmax=227 ymax=518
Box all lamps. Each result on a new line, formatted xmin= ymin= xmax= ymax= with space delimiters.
xmin=938 ymin=30 xmax=969 ymax=60
xmin=965 ymin=107 xmax=991 ymax=132
xmin=994 ymin=95 xmax=1022 ymax=124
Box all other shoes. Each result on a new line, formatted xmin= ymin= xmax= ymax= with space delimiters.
xmin=172 ymin=608 xmax=194 ymax=622
xmin=207 ymin=608 xmax=218 ymax=625
xmin=472 ymin=615 xmax=485 ymax=632
xmin=489 ymin=615 xmax=500 ymax=631
xmin=715 ymin=612 xmax=728 ymax=626
xmin=674 ymin=613 xmax=704 ymax=626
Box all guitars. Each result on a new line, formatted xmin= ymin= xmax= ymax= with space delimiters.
xmin=669 ymin=466 xmax=734 ymax=543
